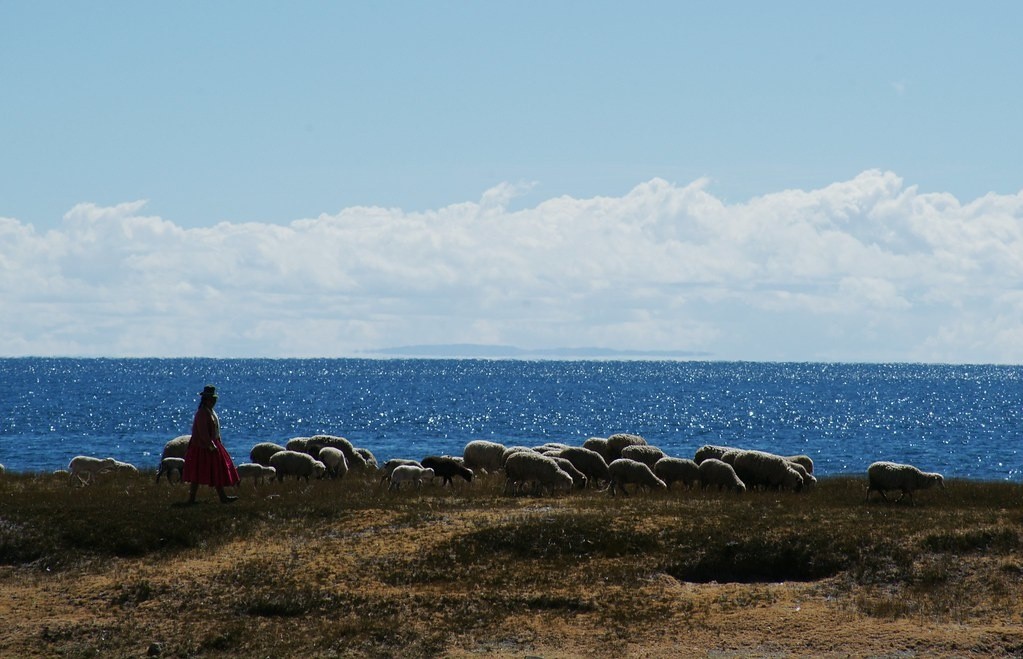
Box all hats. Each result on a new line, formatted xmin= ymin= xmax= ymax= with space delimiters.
xmin=199 ymin=385 xmax=219 ymax=398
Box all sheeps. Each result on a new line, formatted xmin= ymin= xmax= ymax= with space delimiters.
xmin=234 ymin=433 xmax=819 ymax=495
xmin=865 ymin=460 xmax=946 ymax=504
xmin=69 ymin=434 xmax=192 ymax=486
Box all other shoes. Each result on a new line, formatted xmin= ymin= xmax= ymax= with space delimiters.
xmin=220 ymin=496 xmax=239 ymax=503
xmin=185 ymin=500 xmax=200 ymax=506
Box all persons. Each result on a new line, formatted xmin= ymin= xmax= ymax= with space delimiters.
xmin=183 ymin=385 xmax=240 ymax=503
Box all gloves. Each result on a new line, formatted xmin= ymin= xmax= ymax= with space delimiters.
xmin=208 ymin=441 xmax=220 ymax=458
